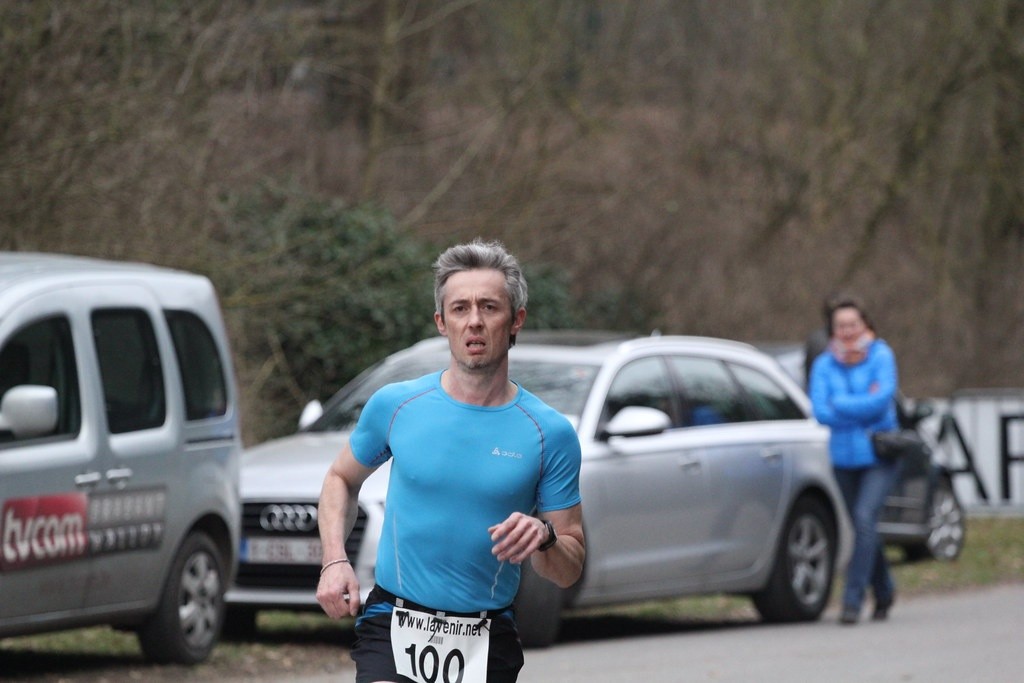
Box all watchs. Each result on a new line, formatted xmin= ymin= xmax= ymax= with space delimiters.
xmin=537 ymin=519 xmax=557 ymax=553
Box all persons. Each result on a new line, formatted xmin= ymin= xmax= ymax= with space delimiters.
xmin=808 ymin=299 xmax=903 ymax=625
xmin=315 ymin=240 xmax=585 ymax=683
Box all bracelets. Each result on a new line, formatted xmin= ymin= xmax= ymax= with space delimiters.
xmin=320 ymin=559 xmax=350 ymax=576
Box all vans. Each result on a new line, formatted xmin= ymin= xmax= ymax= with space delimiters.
xmin=0 ymin=249 xmax=245 ymax=661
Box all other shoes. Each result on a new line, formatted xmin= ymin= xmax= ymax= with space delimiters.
xmin=842 ymin=607 xmax=858 ymax=623
xmin=873 ymin=594 xmax=894 ymax=620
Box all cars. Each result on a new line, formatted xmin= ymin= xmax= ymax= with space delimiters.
xmin=753 ymin=342 xmax=968 ymax=562
xmin=214 ymin=327 xmax=858 ymax=637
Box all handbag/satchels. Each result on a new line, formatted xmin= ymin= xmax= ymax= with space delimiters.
xmin=870 ymin=426 xmax=932 ymax=467
